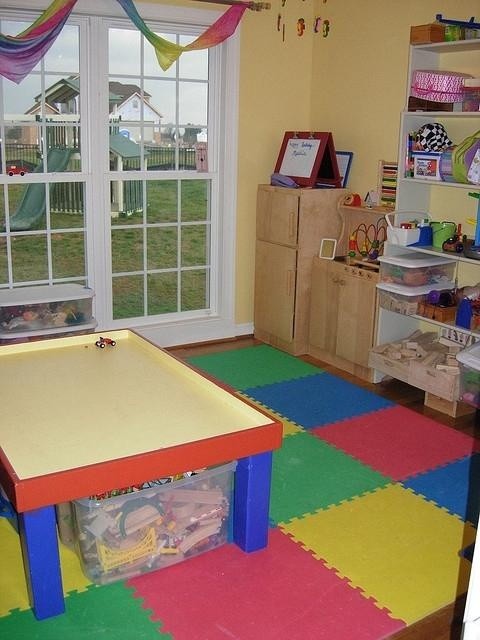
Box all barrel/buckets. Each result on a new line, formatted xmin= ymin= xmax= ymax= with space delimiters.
xmin=431 ymin=222 xmax=456 ymax=248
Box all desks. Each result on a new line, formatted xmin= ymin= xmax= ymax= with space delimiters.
xmin=0 ymin=328 xmax=283 ymax=620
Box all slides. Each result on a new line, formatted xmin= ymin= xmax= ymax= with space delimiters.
xmin=1 ymin=148 xmax=72 ymax=232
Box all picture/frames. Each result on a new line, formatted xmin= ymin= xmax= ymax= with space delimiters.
xmin=413 ymin=154 xmax=442 ymax=181
xmin=319 ymin=238 xmax=337 ymax=260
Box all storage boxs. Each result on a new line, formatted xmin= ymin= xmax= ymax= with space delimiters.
xmin=455 ymin=340 xmax=480 ymax=410
xmin=0 ymin=283 xmax=97 ymax=345
xmin=376 ymin=252 xmax=456 ymax=316
xmin=52 ymin=458 xmax=238 ymax=586
xmin=410 ymin=24 xmax=444 ymax=45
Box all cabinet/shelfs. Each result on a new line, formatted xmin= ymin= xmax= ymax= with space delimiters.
xmin=373 ymin=39 xmax=480 ymax=416
xmin=308 ymin=193 xmax=395 ymax=382
